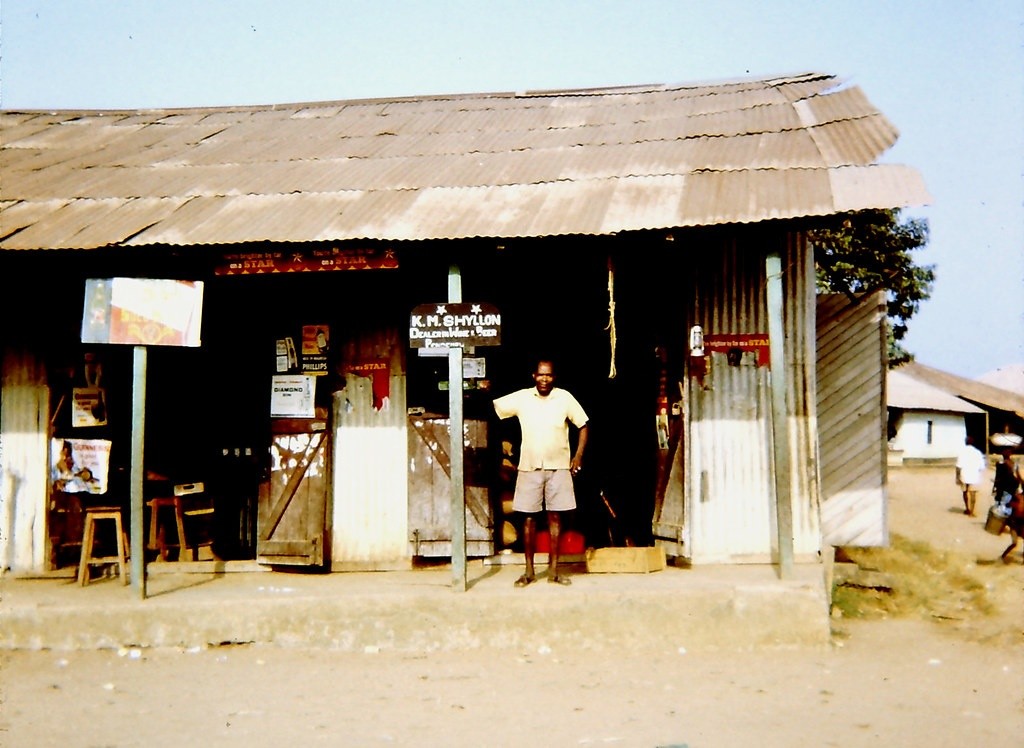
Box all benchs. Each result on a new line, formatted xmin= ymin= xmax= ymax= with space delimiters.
xmin=75 ymin=507 xmax=130 ymax=587
xmin=144 ymin=496 xmax=188 ymax=555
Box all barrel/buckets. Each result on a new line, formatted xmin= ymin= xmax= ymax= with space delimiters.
xmin=985 ymin=505 xmax=1009 ymax=536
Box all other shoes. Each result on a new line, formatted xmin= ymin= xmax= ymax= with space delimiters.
xmin=514 ymin=574 xmax=534 ymax=586
xmin=547 ymin=576 xmax=571 ymax=585
xmin=964 ymin=509 xmax=970 ymax=515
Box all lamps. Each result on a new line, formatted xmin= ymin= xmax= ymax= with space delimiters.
xmin=690 ymin=325 xmax=705 ymax=357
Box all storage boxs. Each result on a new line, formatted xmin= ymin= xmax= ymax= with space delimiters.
xmin=585 ymin=546 xmax=667 ymax=575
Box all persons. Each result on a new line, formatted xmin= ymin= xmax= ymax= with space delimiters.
xmin=956 ymin=437 xmax=985 ymax=516
xmin=1000 ymin=480 xmax=1024 ymax=565
xmin=493 ymin=358 xmax=590 ymax=587
xmin=992 ymin=450 xmax=1024 ymax=505
